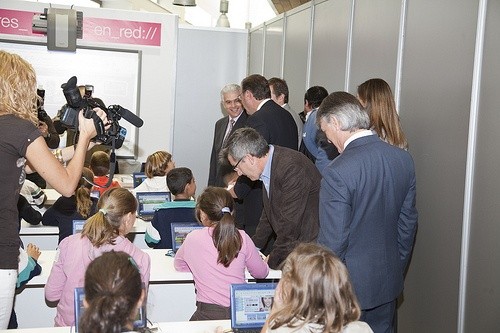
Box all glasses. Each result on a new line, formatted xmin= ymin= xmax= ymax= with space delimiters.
xmin=231 ymin=153 xmax=253 ymax=170
xmin=237 ymin=89 xmax=251 ymax=101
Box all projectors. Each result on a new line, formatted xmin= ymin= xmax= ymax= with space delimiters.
xmin=32 ymin=8 xmax=78 ymax=53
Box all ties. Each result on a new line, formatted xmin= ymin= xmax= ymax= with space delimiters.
xmin=223 ymin=120 xmax=235 ymax=148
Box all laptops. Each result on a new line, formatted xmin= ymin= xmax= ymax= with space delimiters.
xmin=74 ymin=283 xmax=146 ymax=333
xmin=171 ymin=221 xmax=205 ymax=253
xmin=230 ymin=283 xmax=277 ymax=333
xmin=137 ymin=191 xmax=171 ymax=221
xmin=133 ymin=172 xmax=147 ymax=188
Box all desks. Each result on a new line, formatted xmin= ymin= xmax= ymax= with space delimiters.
xmin=0 ymin=173 xmax=283 ymax=333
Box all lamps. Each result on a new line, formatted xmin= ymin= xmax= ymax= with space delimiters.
xmin=173 ymin=0 xmax=196 ymax=5
xmin=217 ymin=0 xmax=231 ymax=28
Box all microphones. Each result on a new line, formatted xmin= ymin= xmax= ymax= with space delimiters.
xmin=114 ymin=105 xmax=143 ymax=127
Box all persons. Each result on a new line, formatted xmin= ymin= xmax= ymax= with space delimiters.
xmin=226 ymin=128 xmax=322 ymax=272
xmin=77 ymin=249 xmax=155 ymax=333
xmin=173 ymin=188 xmax=270 ymax=322
xmin=24 ymin=98 xmax=60 ymax=189
xmin=16 ymin=243 xmax=42 ymax=290
xmin=51 ymin=86 xmax=106 ymax=148
xmin=357 ymin=78 xmax=410 ymax=152
xmin=0 ymin=49 xmax=112 ymax=330
xmin=260 ymin=242 xmax=374 ymax=333
xmin=127 ymin=151 xmax=195 ymax=201
xmin=89 ymin=150 xmax=122 ymax=198
xmin=42 ymin=166 xmax=100 ymax=246
xmin=262 ymin=297 xmax=274 ymax=310
xmin=45 ymin=188 xmax=152 ymax=327
xmin=17 ymin=191 xmax=42 ymax=234
xmin=19 ymin=179 xmax=48 ymax=217
xmin=144 ymin=167 xmax=203 ymax=249
xmin=317 ymin=91 xmax=419 ymax=333
xmin=207 ymin=74 xmax=340 ymax=239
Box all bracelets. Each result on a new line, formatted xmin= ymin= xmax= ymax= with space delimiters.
xmin=49 ymin=150 xmax=64 ymax=165
